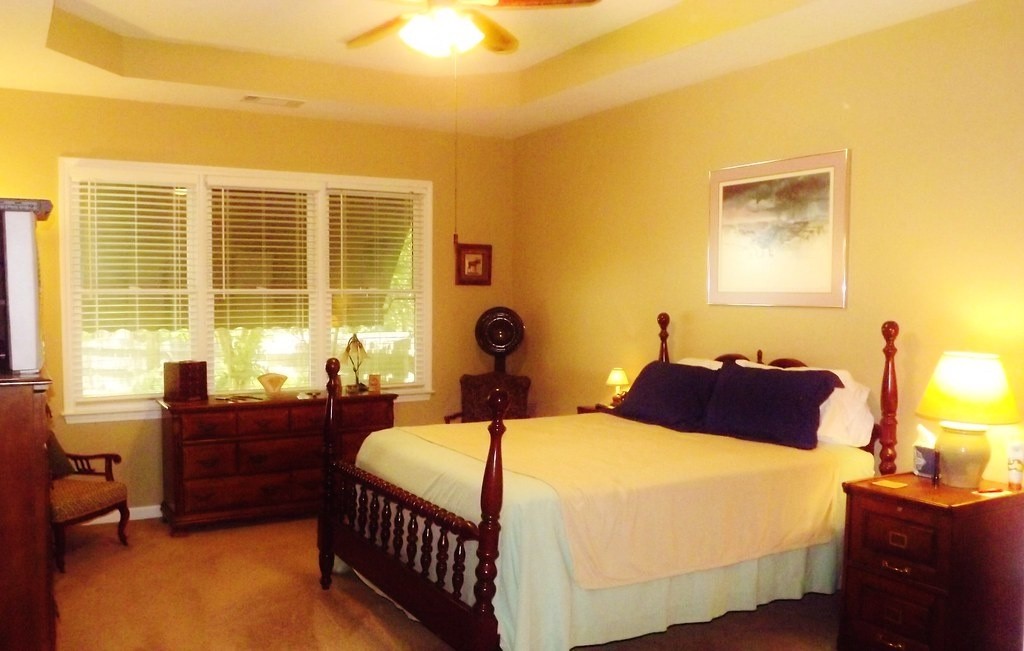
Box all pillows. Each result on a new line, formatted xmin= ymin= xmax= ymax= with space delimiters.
xmin=688 ymin=359 xmax=845 ymax=451
xmin=676 ymin=357 xmax=723 ymax=371
xmin=735 ymin=359 xmax=874 ymax=448
xmin=612 ymin=359 xmax=719 ymax=435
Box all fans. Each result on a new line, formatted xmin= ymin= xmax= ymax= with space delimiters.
xmin=342 ymin=0 xmax=602 ymax=56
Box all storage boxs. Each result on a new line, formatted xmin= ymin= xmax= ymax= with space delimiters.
xmin=163 ymin=361 xmax=209 ymax=401
xmin=369 ymin=374 xmax=381 ymax=393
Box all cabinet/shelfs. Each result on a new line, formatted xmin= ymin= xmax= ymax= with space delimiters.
xmin=154 ymin=387 xmax=400 ymax=534
xmin=0 ymin=198 xmax=53 ymax=651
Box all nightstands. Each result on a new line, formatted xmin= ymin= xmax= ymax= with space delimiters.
xmin=577 ymin=406 xmax=617 ymax=414
xmin=836 ymin=473 xmax=1024 ymax=651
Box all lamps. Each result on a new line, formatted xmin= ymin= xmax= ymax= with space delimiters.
xmin=606 ymin=367 xmax=630 ymax=394
xmin=397 ymin=4 xmax=484 ymax=60
xmin=914 ymin=348 xmax=1022 ymax=491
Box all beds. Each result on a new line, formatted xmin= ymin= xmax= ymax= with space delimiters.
xmin=316 ymin=313 xmax=902 ymax=651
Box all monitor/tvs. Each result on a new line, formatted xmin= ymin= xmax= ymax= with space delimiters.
xmin=0 ymin=210 xmax=45 ymax=375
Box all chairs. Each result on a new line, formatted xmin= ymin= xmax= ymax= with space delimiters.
xmin=444 ymin=372 xmax=531 ymax=424
xmin=45 ymin=404 xmax=132 ymax=575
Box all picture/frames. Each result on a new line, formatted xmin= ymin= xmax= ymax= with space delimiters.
xmin=707 ymin=148 xmax=849 ymax=310
xmin=455 ymin=242 xmax=493 ymax=286
